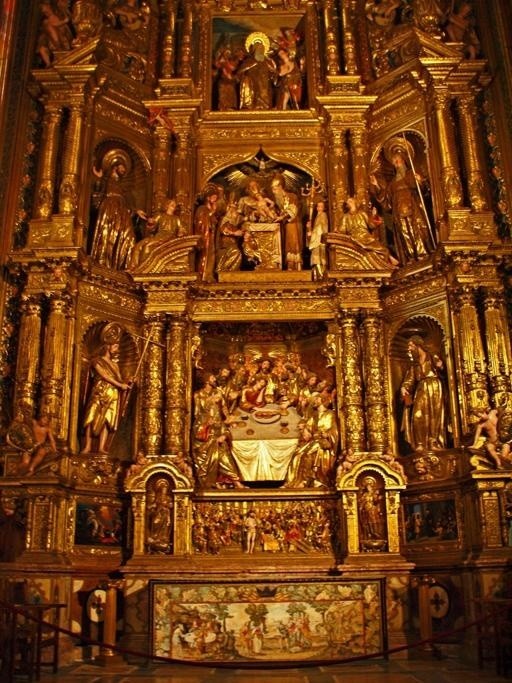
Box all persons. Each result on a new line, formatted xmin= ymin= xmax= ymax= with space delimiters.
xmin=21 ymin=408 xmax=57 ymax=472
xmin=467 ymin=407 xmax=510 ymax=470
xmin=215 ymin=28 xmax=304 ymax=111
xmin=192 ymin=504 xmax=336 ymax=555
xmin=80 ymin=321 xmax=139 ymax=456
xmin=190 ymin=352 xmax=340 ymax=490
xmin=90 ymin=151 xmax=437 ymax=283
xmin=397 ymin=336 xmax=446 ymax=452
xmin=146 ymin=482 xmax=173 ymax=551
xmin=357 ymin=479 xmax=386 ymax=539
